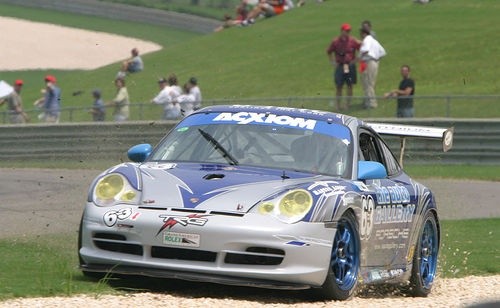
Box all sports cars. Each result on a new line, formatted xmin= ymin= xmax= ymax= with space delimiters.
xmin=78 ymin=102 xmax=454 ymax=301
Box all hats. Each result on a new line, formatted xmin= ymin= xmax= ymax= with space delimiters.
xmin=342 ymin=23 xmax=351 ymax=31
xmin=15 ymin=80 xmax=23 ymax=85
xmin=158 ymin=78 xmax=166 ymax=83
xmin=45 ymin=75 xmax=56 ymax=84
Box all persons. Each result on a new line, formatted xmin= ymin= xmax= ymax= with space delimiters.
xmin=151 ymin=77 xmax=181 ymax=119
xmin=1 ymin=79 xmax=31 ymax=124
xmin=358 ymin=18 xmax=378 ymax=39
xmin=212 ymin=0 xmax=323 ymax=31
xmin=116 ymin=47 xmax=144 ymax=79
xmin=105 ymin=77 xmax=130 ymax=122
xmin=305 ymin=130 xmax=343 ymax=172
xmin=169 ymin=73 xmax=183 ymax=97
xmin=87 ymin=91 xmax=106 ymax=121
xmin=326 ymin=23 xmax=362 ymax=112
xmin=384 ymin=65 xmax=416 ymax=117
xmin=32 ymin=75 xmax=61 ymax=124
xmin=187 ymin=77 xmax=203 ymax=112
xmin=356 ymin=28 xmax=386 ymax=109
xmin=171 ymin=84 xmax=195 ymax=117
